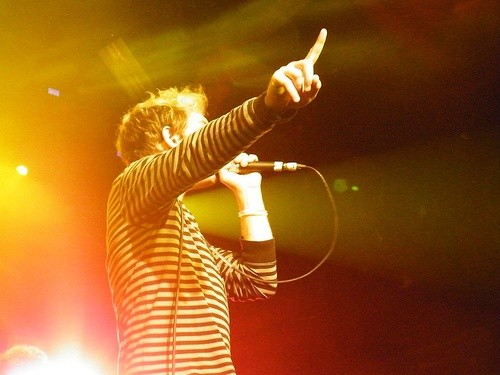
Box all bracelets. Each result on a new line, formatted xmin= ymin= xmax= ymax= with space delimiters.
xmin=238 ymin=207 xmax=270 ymax=219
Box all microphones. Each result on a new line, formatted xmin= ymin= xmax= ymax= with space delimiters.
xmin=217 ymin=161 xmax=306 ymax=180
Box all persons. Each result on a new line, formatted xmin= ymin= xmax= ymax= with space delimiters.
xmin=107 ymin=27 xmax=331 ymax=375
